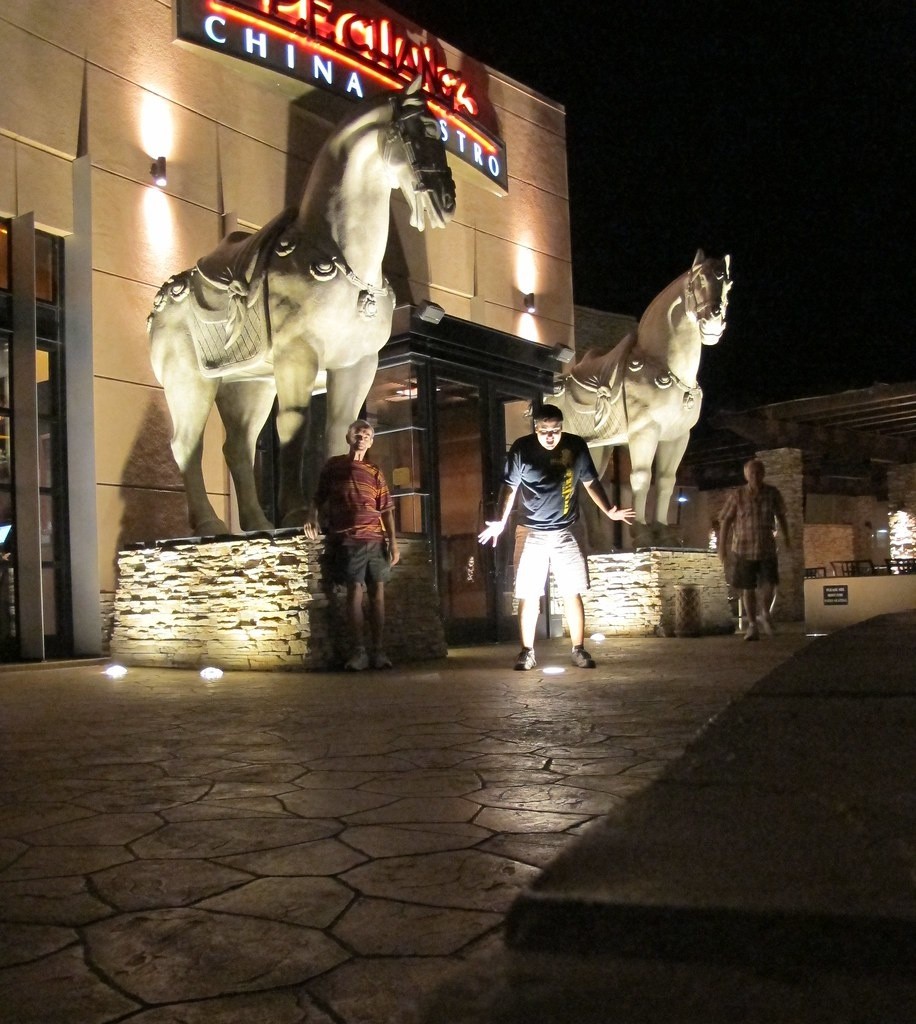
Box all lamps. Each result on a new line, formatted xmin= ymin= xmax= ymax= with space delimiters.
xmin=524 ymin=293 xmax=535 ymax=313
xmin=548 ymin=343 xmax=575 ymax=363
xmin=150 ymin=157 xmax=166 ymax=186
xmin=412 ymin=299 xmax=445 ymax=324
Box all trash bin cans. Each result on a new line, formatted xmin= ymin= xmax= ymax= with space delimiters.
xmin=674 ymin=582 xmax=706 ymax=636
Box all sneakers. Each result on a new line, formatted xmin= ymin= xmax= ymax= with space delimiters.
xmin=760 ymin=611 xmax=777 ymax=636
xmin=370 ymin=651 xmax=392 ymax=670
xmin=570 ymin=648 xmax=595 ymax=668
xmin=744 ymin=625 xmax=760 ymax=641
xmin=347 ymin=653 xmax=368 ymax=671
xmin=513 ymin=648 xmax=537 ymax=671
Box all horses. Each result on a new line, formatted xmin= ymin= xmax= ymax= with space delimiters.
xmin=145 ymin=73 xmax=456 ymax=536
xmin=523 ymin=249 xmax=734 ymax=548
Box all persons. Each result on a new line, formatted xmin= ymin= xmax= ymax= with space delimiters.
xmin=716 ymin=458 xmax=794 ymax=641
xmin=304 ymin=419 xmax=400 ymax=671
xmin=479 ymin=404 xmax=636 ymax=671
